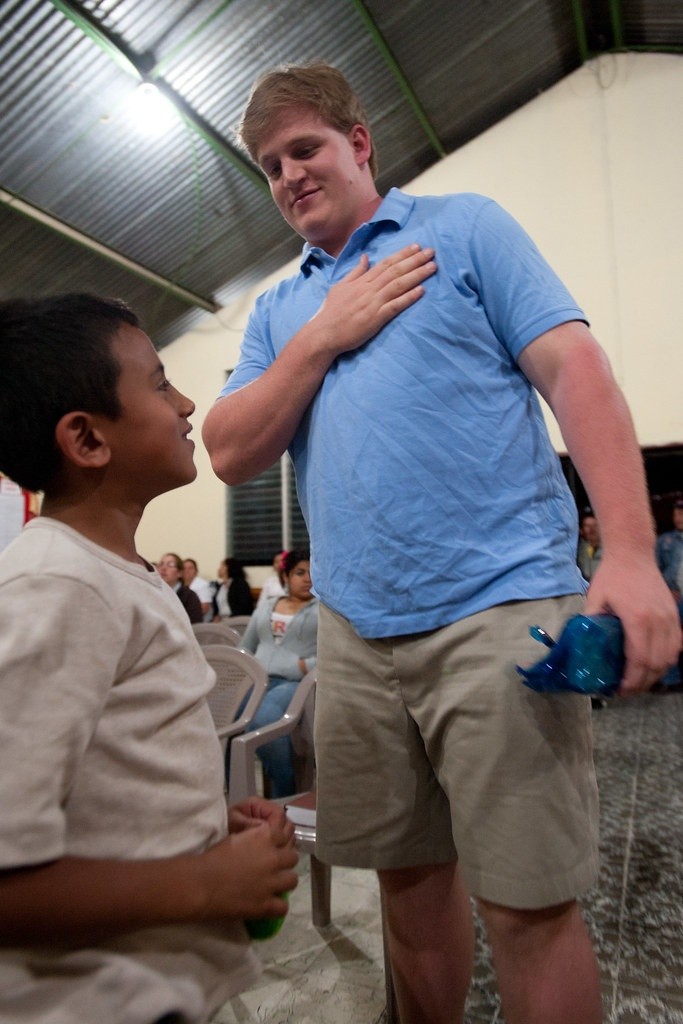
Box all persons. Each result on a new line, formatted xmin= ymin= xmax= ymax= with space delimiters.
xmin=576 ymin=503 xmax=683 ymax=590
xmin=0 ymin=290 xmax=303 ymax=1024
xmin=201 ymin=64 xmax=683 ymax=1024
xmin=157 ymin=547 xmax=317 ymax=802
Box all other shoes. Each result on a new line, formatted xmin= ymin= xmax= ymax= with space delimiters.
xmin=647 ymin=680 xmax=683 ymax=696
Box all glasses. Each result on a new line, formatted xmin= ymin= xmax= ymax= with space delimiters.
xmin=158 ymin=560 xmax=179 ymax=568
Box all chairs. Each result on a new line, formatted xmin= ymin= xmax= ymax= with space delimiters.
xmin=190 ymin=614 xmax=332 ymax=928
